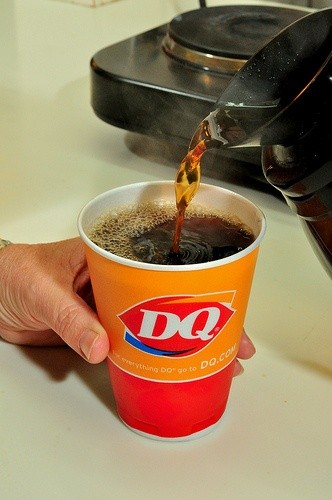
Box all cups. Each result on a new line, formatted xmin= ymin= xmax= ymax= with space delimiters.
xmin=79 ymin=180 xmax=266 ymax=441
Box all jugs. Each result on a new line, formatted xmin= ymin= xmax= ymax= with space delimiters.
xmin=215 ymin=7 xmax=332 ymax=281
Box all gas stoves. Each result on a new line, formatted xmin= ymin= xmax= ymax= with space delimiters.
xmin=91 ymin=0 xmax=316 ymax=166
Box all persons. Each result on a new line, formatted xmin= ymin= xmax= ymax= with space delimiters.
xmin=0 ymin=237 xmax=255 ymax=377
